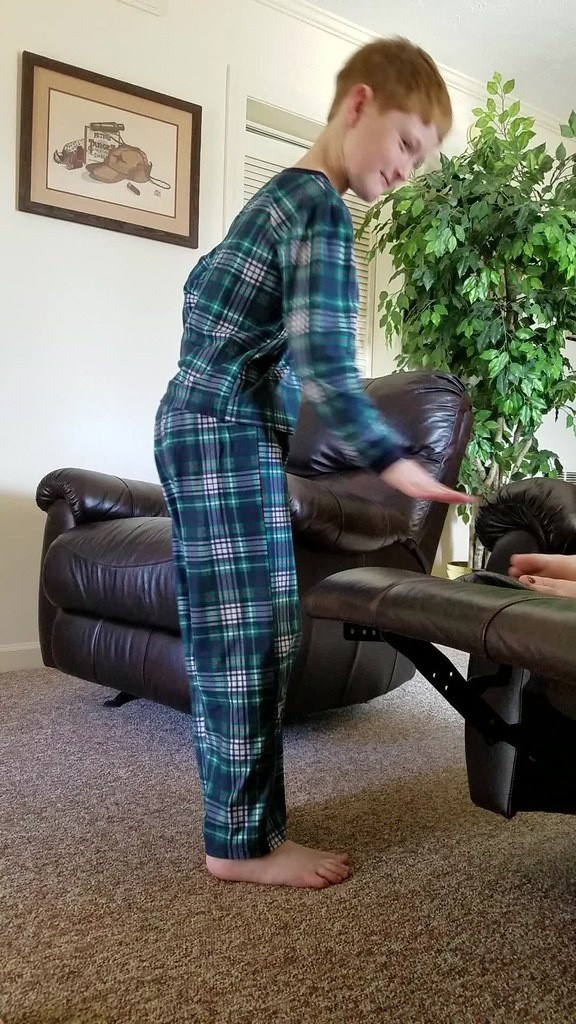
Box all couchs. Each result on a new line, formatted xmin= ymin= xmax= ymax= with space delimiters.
xmin=32 ymin=364 xmax=576 ymax=822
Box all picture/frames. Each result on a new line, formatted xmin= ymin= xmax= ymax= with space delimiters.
xmin=13 ymin=48 xmax=204 ymax=250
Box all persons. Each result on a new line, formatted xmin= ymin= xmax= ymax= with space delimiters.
xmin=152 ymin=37 xmax=480 ymax=889
xmin=508 ymin=553 xmax=576 ymax=596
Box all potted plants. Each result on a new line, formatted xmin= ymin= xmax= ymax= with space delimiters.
xmin=355 ymin=64 xmax=576 ymax=581
